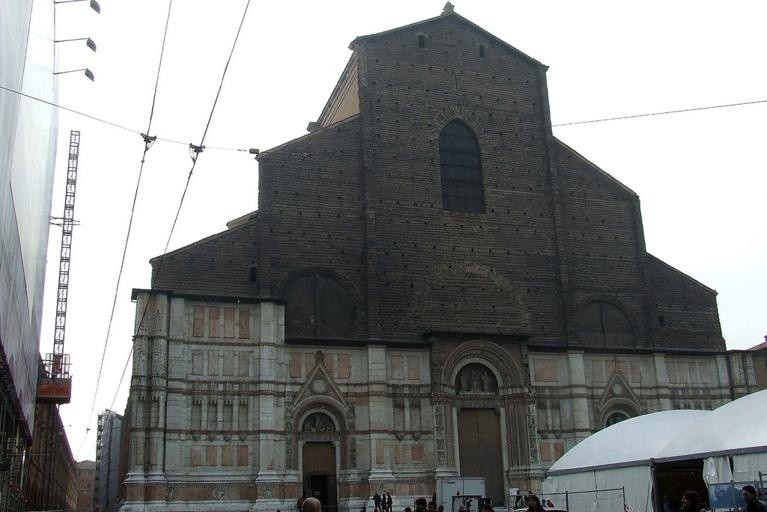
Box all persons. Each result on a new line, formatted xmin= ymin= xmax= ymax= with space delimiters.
xmin=739 ymin=485 xmax=766 ymax=511
xmin=677 ymin=490 xmax=711 ymax=512
xmin=275 ymin=484 xmax=559 ymax=512
